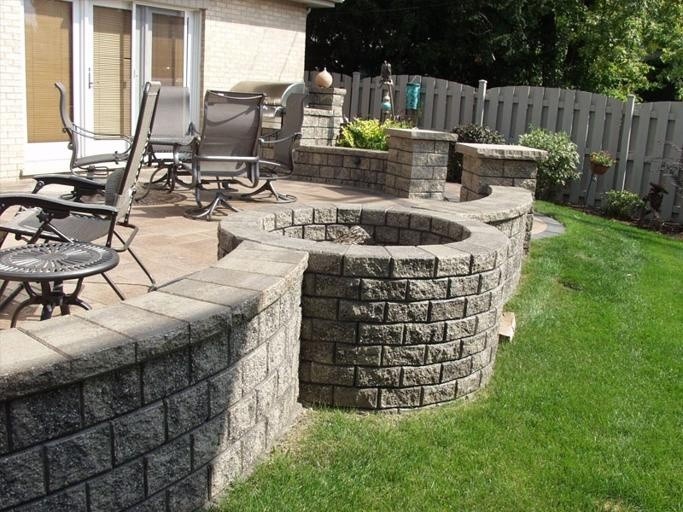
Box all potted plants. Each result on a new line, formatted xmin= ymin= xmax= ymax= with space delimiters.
xmin=590 ymin=151 xmax=613 ymax=174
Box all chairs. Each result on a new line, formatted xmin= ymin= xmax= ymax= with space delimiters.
xmin=140 ymin=82 xmax=302 ymax=220
xmin=1 ymin=81 xmax=161 ymax=300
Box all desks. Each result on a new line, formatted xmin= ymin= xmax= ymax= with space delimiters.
xmin=0 ymin=241 xmax=122 ymax=324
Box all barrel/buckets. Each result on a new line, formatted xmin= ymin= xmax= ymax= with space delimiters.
xmin=405 ymin=74 xmax=422 ymax=110
